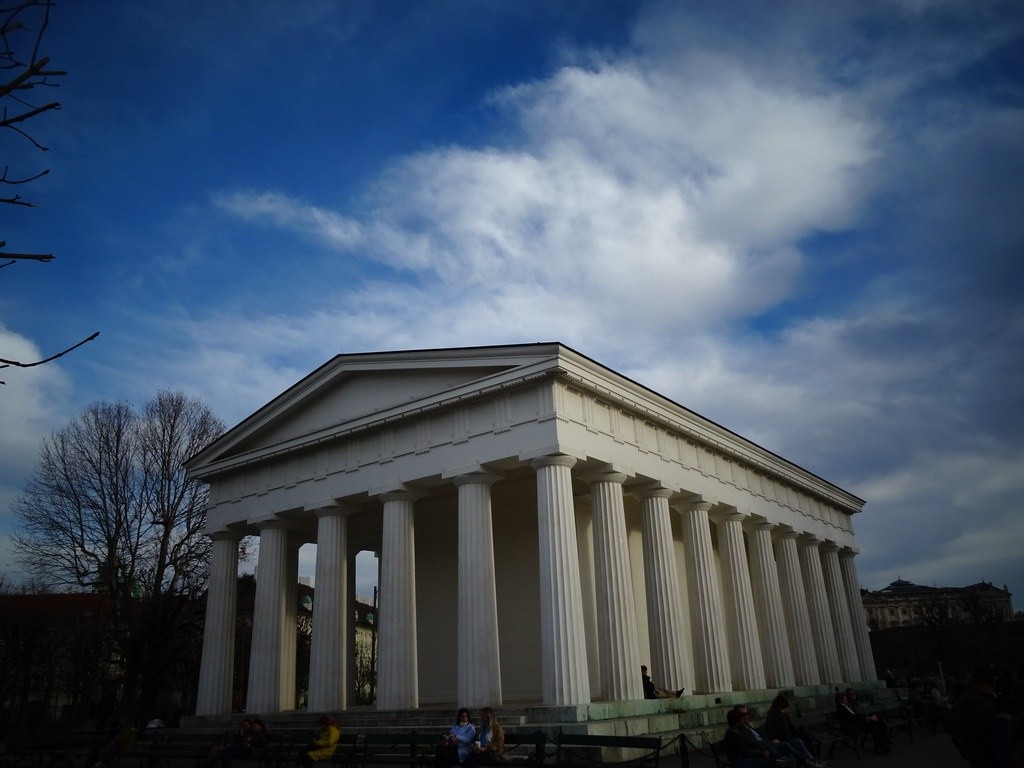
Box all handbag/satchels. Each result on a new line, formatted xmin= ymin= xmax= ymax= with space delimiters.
xmin=494 ymin=753 xmax=513 ymax=765
xmin=435 ymin=744 xmax=460 ymax=766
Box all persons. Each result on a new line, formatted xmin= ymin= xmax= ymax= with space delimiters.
xmin=724 ymin=710 xmax=777 ymax=768
xmin=906 ymin=680 xmax=955 ymax=733
xmin=833 ymin=688 xmax=896 ymax=756
xmin=764 ymin=696 xmax=828 ymax=768
xmin=436 ymin=708 xmax=476 ymax=768
xmin=82 ymin=707 xmax=169 ymax=768
xmin=293 ymin=715 xmax=343 ymax=768
xmin=473 ymin=706 xmax=504 ymax=768
xmin=200 ymin=718 xmax=269 ymax=768
xmin=734 ymin=704 xmax=798 ymax=768
xmin=640 ymin=665 xmax=685 ymax=699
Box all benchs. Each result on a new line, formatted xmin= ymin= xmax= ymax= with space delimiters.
xmin=457 ymin=733 xmax=547 ymax=768
xmin=352 ymin=733 xmax=442 ymax=768
xmin=706 ymin=698 xmax=936 ymax=768
xmin=553 ymin=733 xmax=663 ymax=768
xmin=0 ymin=732 xmax=356 ymax=768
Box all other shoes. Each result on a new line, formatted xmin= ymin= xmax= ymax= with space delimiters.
xmin=675 ymin=688 xmax=684 ymax=697
xmin=807 ymin=758 xmax=827 ymax=768
xmin=874 ymin=745 xmax=893 ymax=755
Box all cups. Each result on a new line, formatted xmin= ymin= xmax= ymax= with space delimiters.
xmin=475 ymin=741 xmax=481 ymax=749
